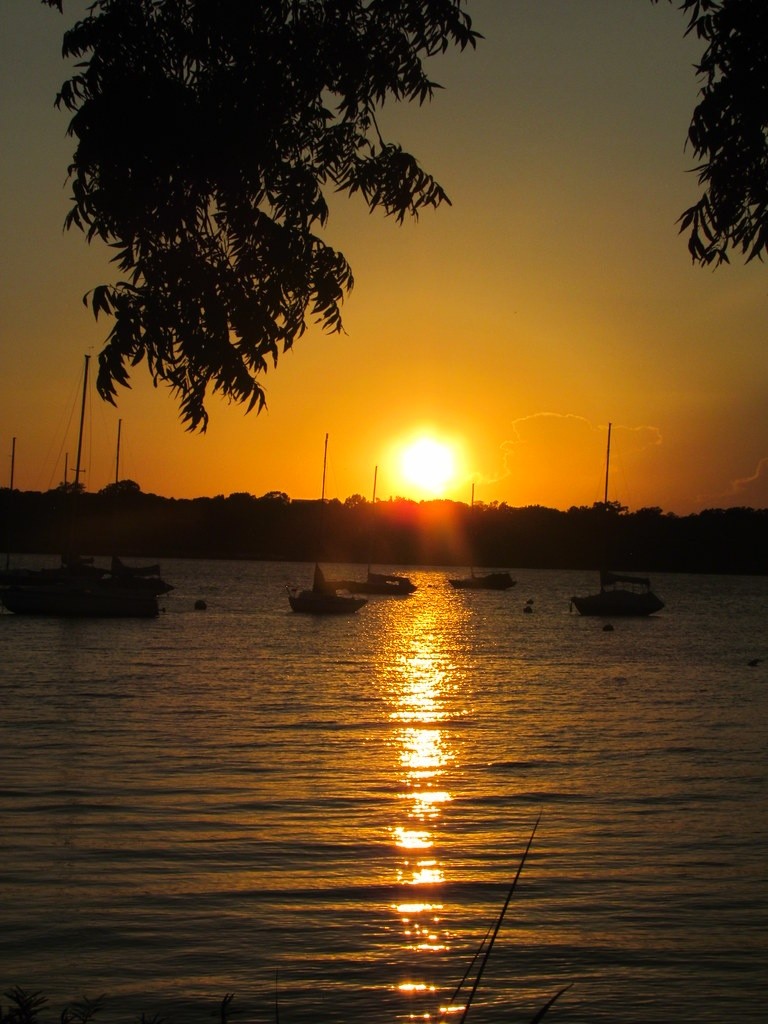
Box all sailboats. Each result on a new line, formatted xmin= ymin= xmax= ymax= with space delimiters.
xmin=446 ymin=484 xmax=516 ymax=591
xmin=1 ymin=352 xmax=173 ymax=621
xmin=288 ymin=431 xmax=368 ymax=616
xmin=570 ymin=424 xmax=664 ymax=618
xmin=350 ymin=464 xmax=418 ymax=594
